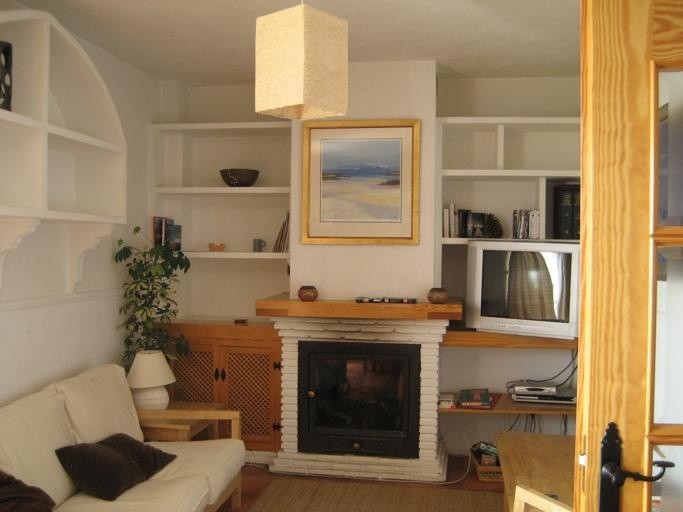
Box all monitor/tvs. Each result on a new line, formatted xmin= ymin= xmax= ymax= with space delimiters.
xmin=465 ymin=239 xmax=579 ymax=341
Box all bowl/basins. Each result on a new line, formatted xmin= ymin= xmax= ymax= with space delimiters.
xmin=218 ymin=169 xmax=259 ymax=187
xmin=208 ymin=243 xmax=224 ymax=251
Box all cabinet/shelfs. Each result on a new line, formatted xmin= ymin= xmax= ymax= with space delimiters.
xmin=435 ymin=116 xmax=581 ymax=247
xmin=439 ymin=327 xmax=579 ymax=417
xmin=2 ymin=8 xmax=130 ymax=224
xmin=155 ymin=315 xmax=283 ymax=452
xmin=143 ymin=120 xmax=290 ymax=262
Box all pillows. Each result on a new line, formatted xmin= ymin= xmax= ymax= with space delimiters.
xmin=57 ymin=432 xmax=176 ymax=502
xmin=2 ymin=469 xmax=58 ymax=512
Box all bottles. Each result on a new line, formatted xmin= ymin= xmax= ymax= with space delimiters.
xmin=512 ymin=209 xmax=529 ymax=239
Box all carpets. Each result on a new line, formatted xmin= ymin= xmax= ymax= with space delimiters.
xmin=249 ymin=477 xmax=506 ymax=511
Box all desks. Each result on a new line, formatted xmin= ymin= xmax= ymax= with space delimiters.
xmin=139 ymin=403 xmax=226 ymax=444
xmin=492 ymin=426 xmax=575 ymax=511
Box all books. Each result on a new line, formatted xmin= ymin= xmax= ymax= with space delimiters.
xmin=442 ymin=203 xmax=490 ymax=238
xmin=510 ymin=210 xmax=541 ymax=240
xmin=271 ymin=211 xmax=291 ymax=254
xmin=440 ymin=388 xmax=494 ymax=409
xmin=152 ymin=215 xmax=183 ymax=253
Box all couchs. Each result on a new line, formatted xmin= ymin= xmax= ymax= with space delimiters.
xmin=1 ymin=361 xmax=245 ymax=510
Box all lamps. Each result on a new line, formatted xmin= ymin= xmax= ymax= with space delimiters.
xmin=254 ymin=1 xmax=350 ymax=122
xmin=127 ymin=349 xmax=179 ymax=413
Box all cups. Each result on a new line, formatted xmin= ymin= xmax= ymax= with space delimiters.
xmin=252 ymin=238 xmax=266 ymax=251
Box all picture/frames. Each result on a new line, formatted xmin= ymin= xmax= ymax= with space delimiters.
xmin=301 ymin=116 xmax=422 ymax=247
